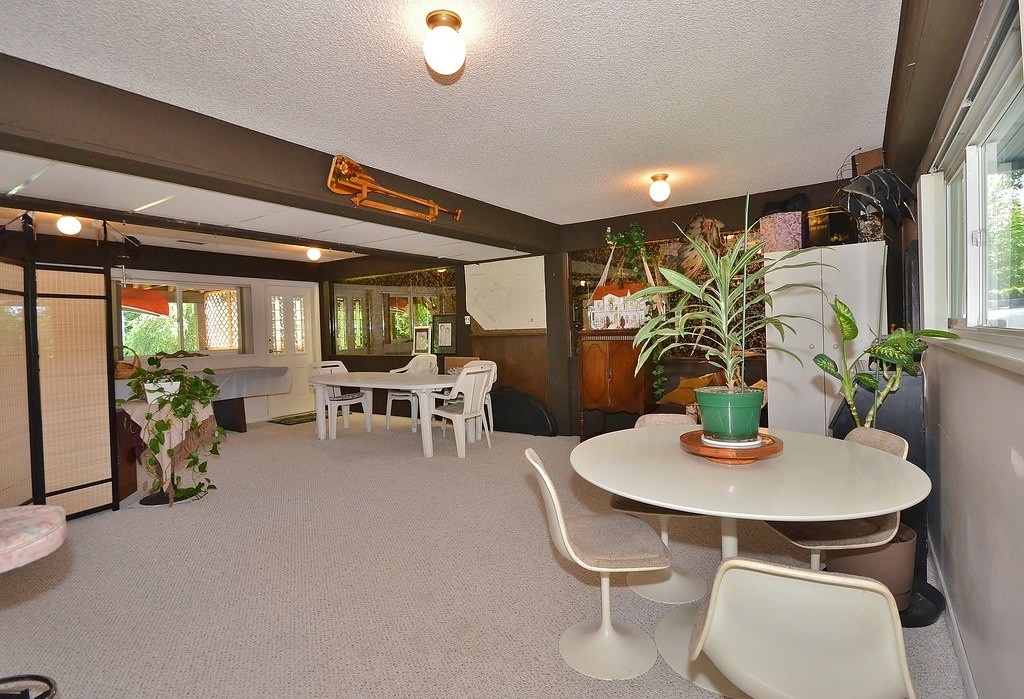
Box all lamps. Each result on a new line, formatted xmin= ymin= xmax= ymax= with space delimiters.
xmin=307 ymin=248 xmax=321 ymax=260
xmin=57 ymin=215 xmax=81 ymax=235
xmin=650 ymin=174 xmax=671 ymax=202
xmin=424 ymin=10 xmax=466 ymax=76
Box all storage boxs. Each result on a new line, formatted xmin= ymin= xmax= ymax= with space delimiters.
xmin=759 ymin=211 xmax=809 ymax=255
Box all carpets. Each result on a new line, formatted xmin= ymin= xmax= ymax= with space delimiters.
xmin=267 ymin=410 xmax=352 ymax=425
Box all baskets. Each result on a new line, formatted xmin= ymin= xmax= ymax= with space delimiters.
xmin=114 ymin=346 xmax=140 ymax=379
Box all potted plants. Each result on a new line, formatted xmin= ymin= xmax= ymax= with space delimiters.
xmin=815 ymin=296 xmax=961 ymax=618
xmin=625 ymin=222 xmax=839 ymax=445
xmin=130 ymin=357 xmax=227 ymax=501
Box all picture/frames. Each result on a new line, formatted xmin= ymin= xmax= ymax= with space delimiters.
xmin=431 ymin=314 xmax=457 ymax=355
xmin=413 ymin=326 xmax=431 ymax=354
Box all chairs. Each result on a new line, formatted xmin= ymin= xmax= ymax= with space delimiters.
xmin=307 ymin=361 xmax=371 ymax=440
xmin=767 ymin=425 xmax=909 ymax=570
xmin=610 ymin=413 xmax=708 ymax=605
xmin=689 ymin=559 xmax=915 ymax=699
xmin=525 ymin=447 xmax=674 ymax=681
xmin=386 ymin=354 xmax=497 ymax=458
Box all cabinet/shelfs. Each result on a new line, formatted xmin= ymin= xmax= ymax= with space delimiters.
xmin=577 ymin=329 xmax=654 ymax=441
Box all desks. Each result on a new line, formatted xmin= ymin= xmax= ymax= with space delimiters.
xmin=308 ymin=372 xmax=475 ymax=457
xmin=184 ymin=366 xmax=292 ymax=432
